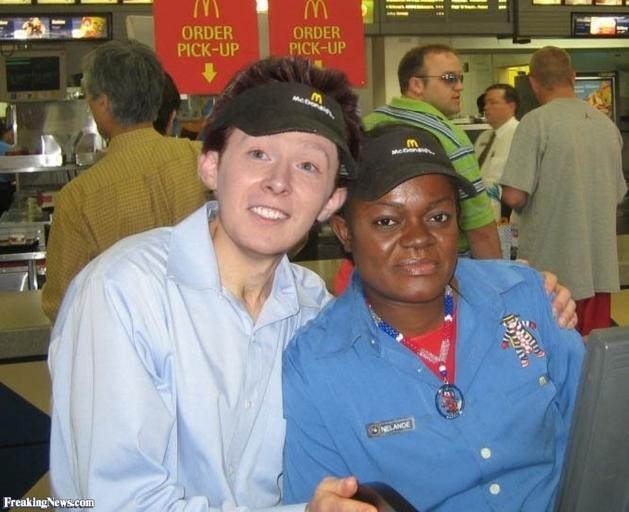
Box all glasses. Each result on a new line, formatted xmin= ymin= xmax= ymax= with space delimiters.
xmin=417 ymin=71 xmax=465 ymax=82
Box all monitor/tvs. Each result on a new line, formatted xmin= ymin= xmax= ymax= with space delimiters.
xmin=0 ymin=49 xmax=68 ymax=104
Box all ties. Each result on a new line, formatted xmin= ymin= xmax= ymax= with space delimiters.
xmin=477 ymin=132 xmax=496 ymax=170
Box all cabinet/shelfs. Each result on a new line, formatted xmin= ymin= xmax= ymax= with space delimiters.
xmin=0 ymin=94 xmax=102 ymax=290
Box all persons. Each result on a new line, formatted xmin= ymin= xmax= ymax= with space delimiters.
xmin=42 ymin=39 xmax=628 ymax=512
xmin=0 ymin=127 xmax=15 ymax=216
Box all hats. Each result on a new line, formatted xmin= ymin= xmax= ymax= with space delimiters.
xmin=202 ymin=81 xmax=478 ymax=201
